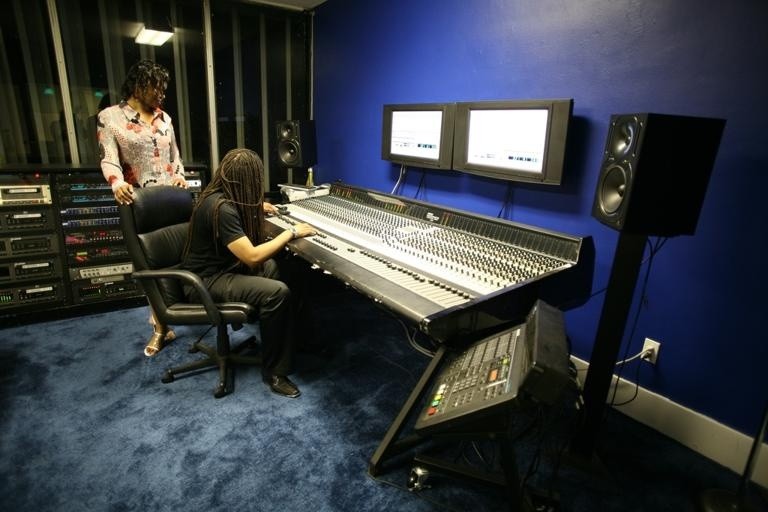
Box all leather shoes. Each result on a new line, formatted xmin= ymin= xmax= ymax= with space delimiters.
xmin=261 ymin=370 xmax=301 ymax=398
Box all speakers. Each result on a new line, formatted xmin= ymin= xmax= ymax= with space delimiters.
xmin=275 ymin=118 xmax=317 ymax=169
xmin=590 ymin=112 xmax=728 ymax=236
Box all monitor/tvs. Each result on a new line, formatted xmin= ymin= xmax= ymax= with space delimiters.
xmin=452 ymin=98 xmax=574 ymax=186
xmin=381 ymin=102 xmax=456 ymax=171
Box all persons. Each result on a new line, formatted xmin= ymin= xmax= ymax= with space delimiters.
xmin=176 ymin=148 xmax=319 ymax=399
xmin=95 ymin=58 xmax=190 ymax=359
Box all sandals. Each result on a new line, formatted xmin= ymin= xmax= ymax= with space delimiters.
xmin=144 ymin=315 xmax=176 ymax=357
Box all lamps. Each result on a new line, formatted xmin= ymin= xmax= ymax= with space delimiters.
xmin=134 ymin=24 xmax=175 ymax=48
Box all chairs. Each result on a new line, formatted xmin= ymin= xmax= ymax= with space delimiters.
xmin=119 ymin=185 xmax=263 ymax=398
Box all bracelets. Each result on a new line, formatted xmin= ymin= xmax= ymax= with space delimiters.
xmin=286 ymin=226 xmax=299 ymax=239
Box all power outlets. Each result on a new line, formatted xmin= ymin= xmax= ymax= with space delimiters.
xmin=640 ymin=338 xmax=661 ymax=364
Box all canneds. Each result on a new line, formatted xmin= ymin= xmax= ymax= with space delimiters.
xmin=407 ymin=466 xmax=430 ymax=493
